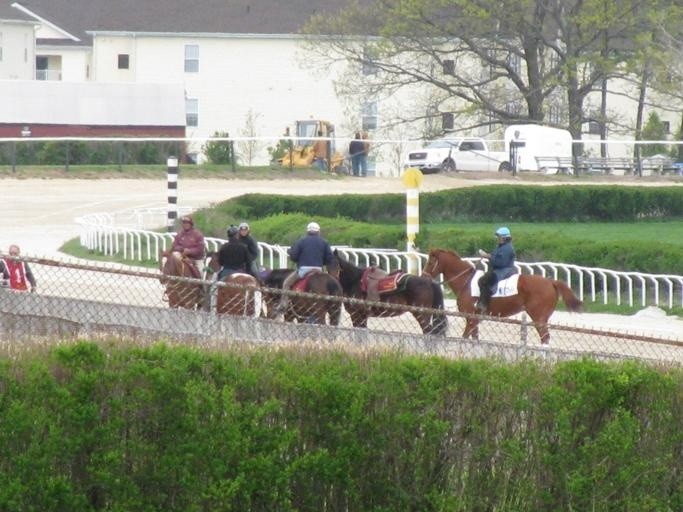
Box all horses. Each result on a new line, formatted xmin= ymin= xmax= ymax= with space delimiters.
xmin=160 ymin=245 xmax=206 ymax=311
xmin=203 ymin=246 xmax=271 ymax=318
xmin=421 ymin=248 xmax=584 ymax=345
xmin=256 ymin=262 xmax=343 ymax=325
xmin=323 ymin=248 xmax=448 ymax=335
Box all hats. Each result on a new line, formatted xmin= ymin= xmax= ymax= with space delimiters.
xmin=227 ymin=225 xmax=240 ymax=236
xmin=182 ymin=216 xmax=193 ymax=225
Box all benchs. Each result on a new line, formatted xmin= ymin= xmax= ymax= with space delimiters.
xmin=534 ymin=155 xmax=680 ymax=176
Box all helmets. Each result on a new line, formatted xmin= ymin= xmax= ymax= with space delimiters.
xmin=239 ymin=223 xmax=250 ymax=232
xmin=495 ymin=227 xmax=512 ymax=240
xmin=307 ymin=223 xmax=320 ymax=232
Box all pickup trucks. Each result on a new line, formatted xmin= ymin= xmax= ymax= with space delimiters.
xmin=405 ymin=134 xmax=515 ymax=175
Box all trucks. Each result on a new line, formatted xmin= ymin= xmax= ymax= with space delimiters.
xmin=505 ymin=124 xmax=577 ymax=176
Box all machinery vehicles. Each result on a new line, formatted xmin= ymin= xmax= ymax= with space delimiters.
xmin=270 ymin=119 xmax=350 ymax=175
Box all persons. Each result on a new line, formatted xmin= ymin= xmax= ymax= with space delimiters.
xmin=4 ymin=245 xmax=37 ymax=292
xmin=349 ymin=133 xmax=364 ymax=176
xmin=218 ymin=224 xmax=246 ymax=279
xmin=478 ymin=227 xmax=516 ymax=311
xmin=238 ymin=223 xmax=263 ymax=284
xmin=314 ymin=131 xmax=327 ymax=170
xmin=361 ymin=133 xmax=369 ymax=176
xmin=171 ymin=216 xmax=207 ymax=281
xmin=288 ymin=222 xmax=335 ymax=279
